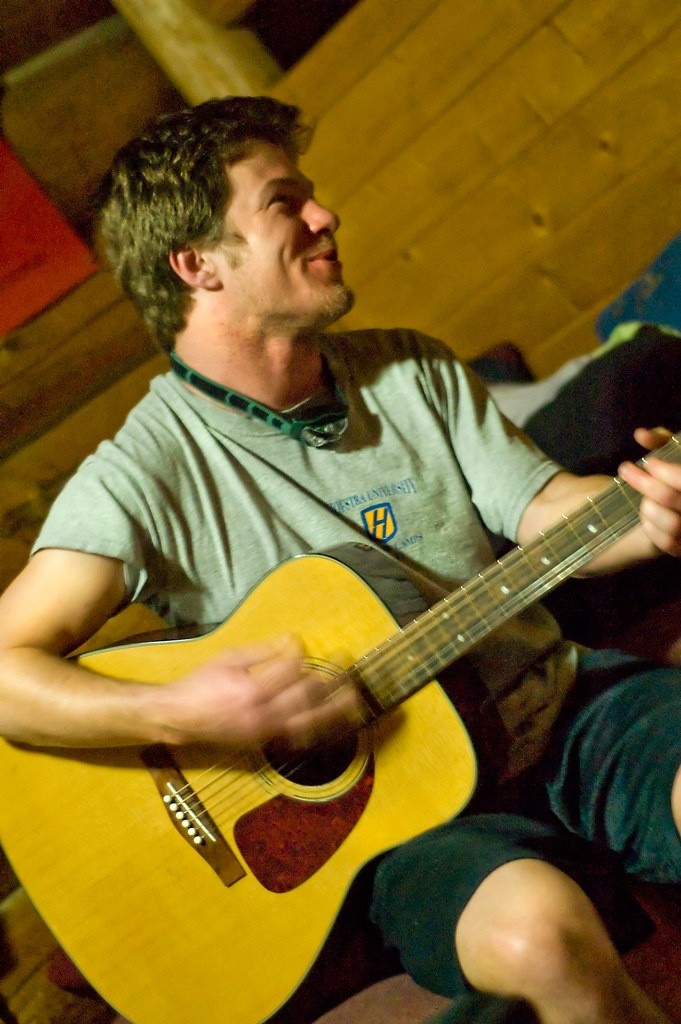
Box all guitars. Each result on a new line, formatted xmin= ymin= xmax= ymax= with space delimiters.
xmin=1 ymin=426 xmax=681 ymax=1024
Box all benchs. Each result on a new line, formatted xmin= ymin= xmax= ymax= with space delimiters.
xmin=0 ymin=850 xmax=473 ymax=1024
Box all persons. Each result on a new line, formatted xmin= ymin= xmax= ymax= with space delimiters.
xmin=0 ymin=96 xmax=681 ymax=1024
